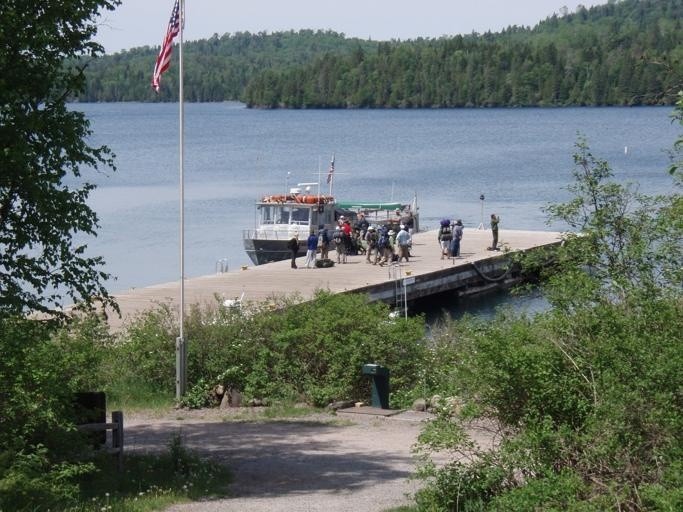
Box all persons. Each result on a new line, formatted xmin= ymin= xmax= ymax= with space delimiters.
xmin=303 ymin=207 xmax=414 ymax=268
xmin=436 ymin=219 xmax=464 ymax=259
xmin=489 ymin=214 xmax=500 ymax=250
xmin=287 ymin=232 xmax=299 ymax=268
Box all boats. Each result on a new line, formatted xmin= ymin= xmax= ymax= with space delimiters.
xmin=241 ymin=152 xmax=420 ymax=266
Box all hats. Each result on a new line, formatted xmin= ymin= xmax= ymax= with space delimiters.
xmin=399 ymin=225 xmax=405 ymax=228
xmin=388 ymin=230 xmax=396 ymax=236
xmin=366 ymin=226 xmax=374 ymax=231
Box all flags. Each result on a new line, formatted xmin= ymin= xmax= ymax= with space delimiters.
xmin=326 ymin=157 xmax=335 ymax=183
xmin=150 ymin=0 xmax=184 ymax=94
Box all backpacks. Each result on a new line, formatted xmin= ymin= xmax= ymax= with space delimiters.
xmin=317 ymin=234 xmax=325 ymax=248
